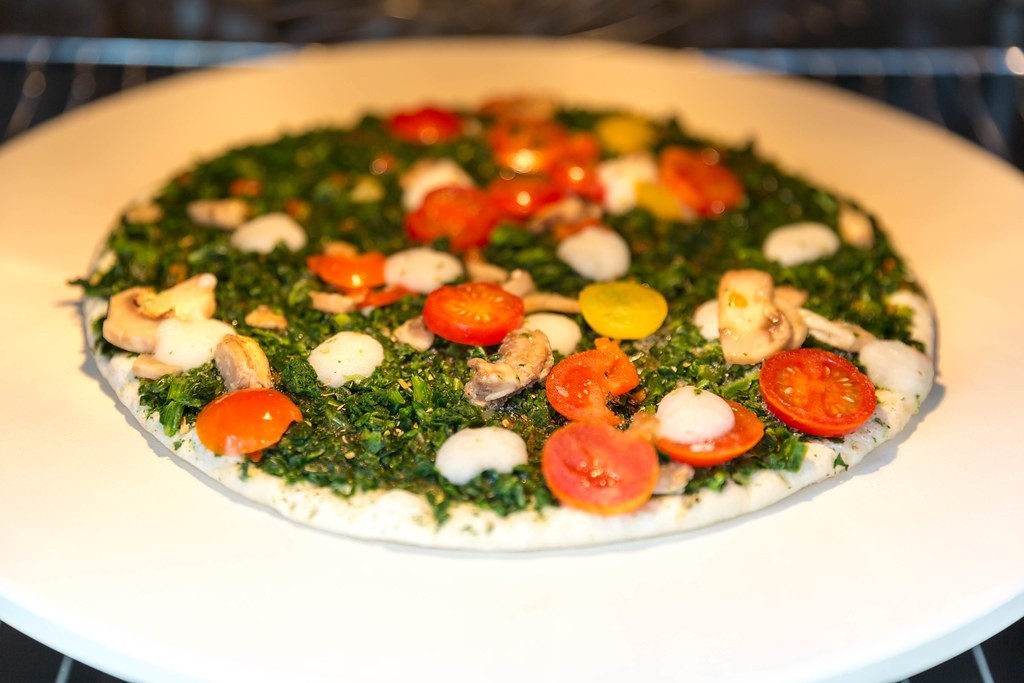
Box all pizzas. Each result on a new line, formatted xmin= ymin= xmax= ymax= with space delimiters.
xmin=69 ymin=91 xmax=936 ymax=547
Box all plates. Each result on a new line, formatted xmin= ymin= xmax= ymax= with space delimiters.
xmin=0 ymin=37 xmax=1024 ymax=683
